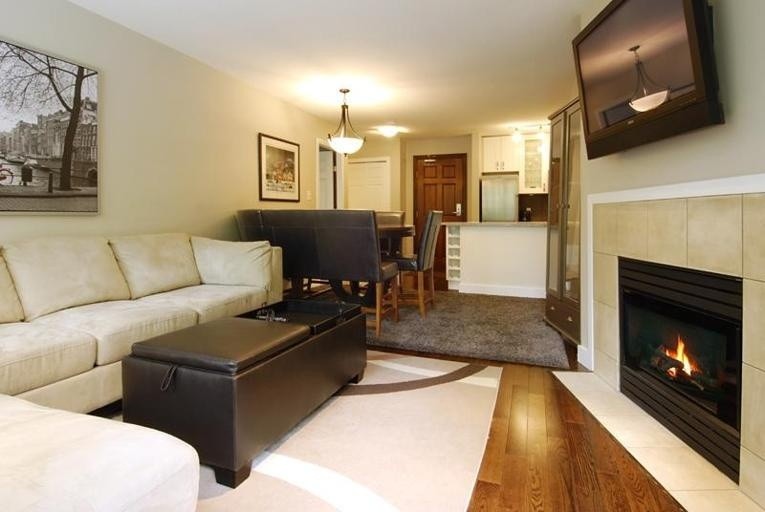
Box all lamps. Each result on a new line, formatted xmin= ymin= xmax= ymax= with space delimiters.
xmin=328 ymin=88 xmax=365 ymax=158
xmin=623 ymin=45 xmax=672 ymax=112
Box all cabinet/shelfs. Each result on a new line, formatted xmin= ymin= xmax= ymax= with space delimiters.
xmin=480 ymin=135 xmax=519 ymax=174
xmin=517 ymin=133 xmax=550 ymax=195
xmin=543 ymin=96 xmax=580 ymax=362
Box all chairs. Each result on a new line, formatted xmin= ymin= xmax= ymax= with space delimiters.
xmin=236 ymin=206 xmax=444 ymax=338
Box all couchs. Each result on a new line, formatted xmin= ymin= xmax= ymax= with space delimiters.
xmin=0 ymin=246 xmax=284 ymax=418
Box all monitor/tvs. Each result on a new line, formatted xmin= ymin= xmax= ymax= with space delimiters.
xmin=570 ymin=0 xmax=725 ymax=160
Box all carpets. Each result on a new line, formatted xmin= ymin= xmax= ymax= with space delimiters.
xmin=291 ymin=283 xmax=570 ymax=368
xmin=107 ymin=349 xmax=503 ymax=512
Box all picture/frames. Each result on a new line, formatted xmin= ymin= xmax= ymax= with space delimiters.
xmin=0 ymin=36 xmax=104 ymax=216
xmin=258 ymin=132 xmax=302 ymax=202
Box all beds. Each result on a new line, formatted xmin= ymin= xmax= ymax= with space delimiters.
xmin=0 ymin=393 xmax=200 ymax=512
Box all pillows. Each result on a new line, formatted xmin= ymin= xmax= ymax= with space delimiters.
xmin=0 ymin=232 xmax=271 ymax=321
